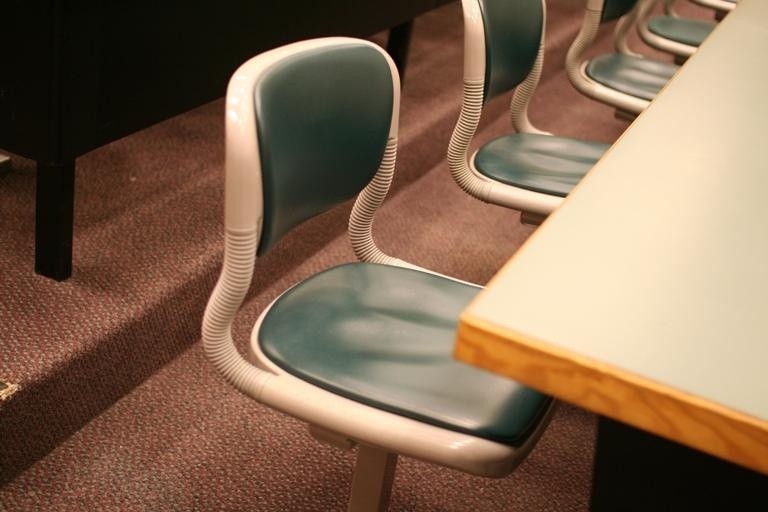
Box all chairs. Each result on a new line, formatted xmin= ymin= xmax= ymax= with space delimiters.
xmin=444 ymin=1 xmax=615 ymax=217
xmin=202 ymin=30 xmax=562 ymax=510
xmin=565 ymin=2 xmax=736 ymax=117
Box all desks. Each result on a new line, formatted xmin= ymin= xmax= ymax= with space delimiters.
xmin=454 ymin=1 xmax=768 ymax=512
